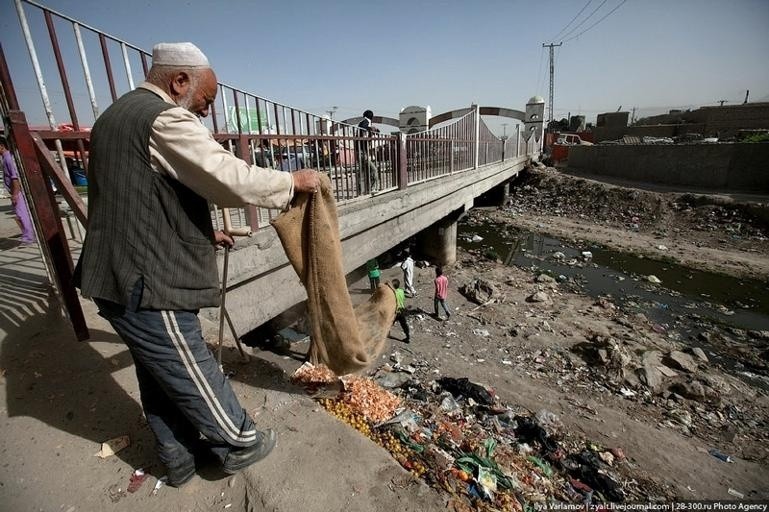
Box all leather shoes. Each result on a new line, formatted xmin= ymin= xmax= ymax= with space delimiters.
xmin=166 ymin=427 xmax=277 ymax=488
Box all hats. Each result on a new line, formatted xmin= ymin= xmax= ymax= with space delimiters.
xmin=152 ymin=42 xmax=210 ymax=70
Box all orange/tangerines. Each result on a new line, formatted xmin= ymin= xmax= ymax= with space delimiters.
xmin=314 ymin=398 xmax=426 ymax=477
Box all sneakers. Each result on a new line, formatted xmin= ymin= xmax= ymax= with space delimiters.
xmin=403 ymin=338 xmax=411 ymax=344
xmin=446 ymin=311 xmax=451 ymax=319
xmin=433 ymin=313 xmax=439 ymax=318
xmin=412 ymin=291 xmax=416 ymax=298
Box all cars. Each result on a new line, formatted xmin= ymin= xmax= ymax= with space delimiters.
xmin=557 ymin=134 xmax=593 ymax=145
xmin=276 ymin=145 xmax=317 ymax=170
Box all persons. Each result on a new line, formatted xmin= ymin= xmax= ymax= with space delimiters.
xmin=353 ymin=110 xmax=381 ymax=196
xmin=399 ymin=250 xmax=418 ymax=299
xmin=430 ymin=267 xmax=451 ymax=319
xmin=391 ymin=278 xmax=411 ymax=343
xmin=77 ymin=39 xmax=326 ymax=487
xmin=366 ymin=258 xmax=381 ymax=295
xmin=0 ymin=136 xmax=37 ymax=242
